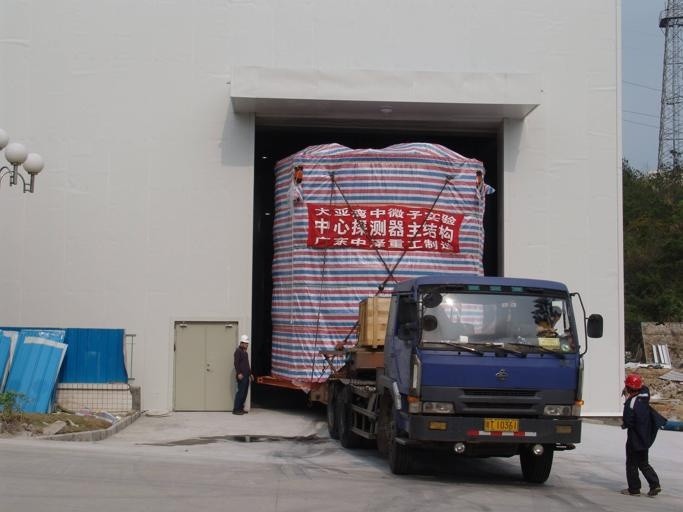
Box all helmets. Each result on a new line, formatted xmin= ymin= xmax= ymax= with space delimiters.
xmin=239 ymin=334 xmax=250 ymax=343
xmin=624 ymin=374 xmax=643 ymax=390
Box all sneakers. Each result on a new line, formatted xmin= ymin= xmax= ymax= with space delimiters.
xmin=647 ymin=485 xmax=661 ymax=497
xmin=620 ymin=489 xmax=641 ymax=496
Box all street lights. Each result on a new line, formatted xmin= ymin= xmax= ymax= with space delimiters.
xmin=0 ymin=126 xmax=43 ymax=194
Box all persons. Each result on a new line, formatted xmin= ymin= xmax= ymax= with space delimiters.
xmin=534 ymin=305 xmax=564 ymax=339
xmin=232 ymin=334 xmax=255 ymax=413
xmin=618 ymin=374 xmax=668 ymax=496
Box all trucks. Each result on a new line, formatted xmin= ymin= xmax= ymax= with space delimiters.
xmin=320 ymin=267 xmax=606 ymax=488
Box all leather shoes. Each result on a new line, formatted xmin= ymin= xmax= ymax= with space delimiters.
xmin=241 ymin=410 xmax=248 ymax=413
xmin=232 ymin=410 xmax=244 ymax=414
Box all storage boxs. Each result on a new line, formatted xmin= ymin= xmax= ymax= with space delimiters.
xmin=356 ymin=296 xmax=391 ymax=350
